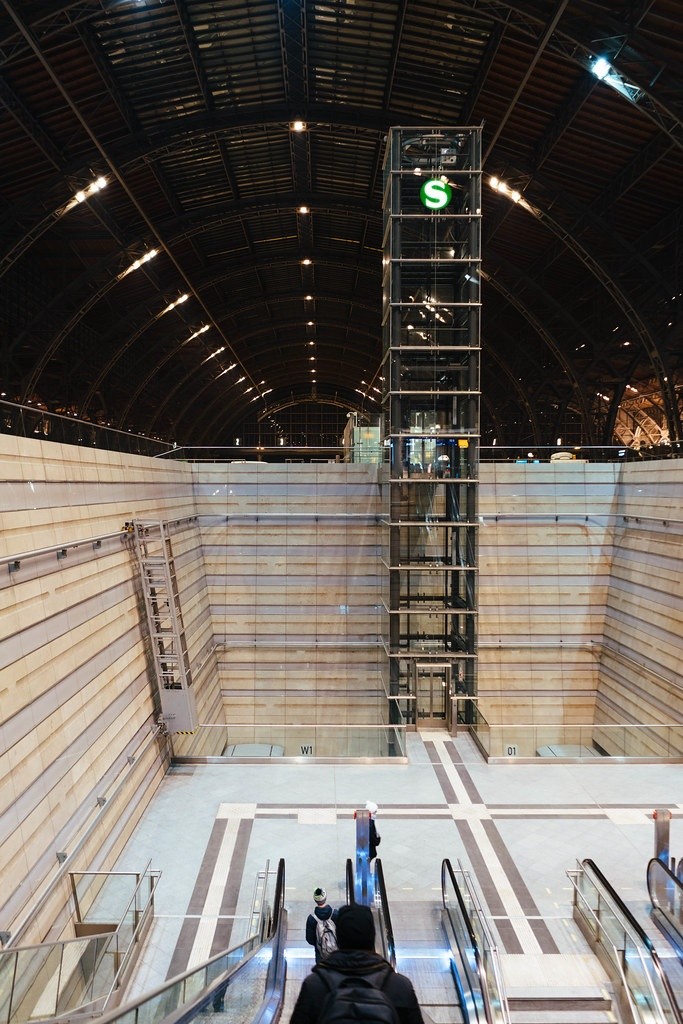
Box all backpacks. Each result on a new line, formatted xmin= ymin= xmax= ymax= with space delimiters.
xmin=311 ymin=963 xmax=400 ymax=1024
xmin=310 ymin=908 xmax=339 ymax=959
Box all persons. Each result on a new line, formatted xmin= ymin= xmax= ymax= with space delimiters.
xmin=305 ymin=888 xmax=339 ymax=965
xmin=289 ymin=904 xmax=425 ymax=1024
xmin=365 ymin=800 xmax=381 ymax=863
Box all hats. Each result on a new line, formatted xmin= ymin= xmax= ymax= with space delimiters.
xmin=312 ymin=888 xmax=327 ymax=904
xmin=365 ymin=801 xmax=378 ymax=813
xmin=335 ymin=903 xmax=375 ymax=950
